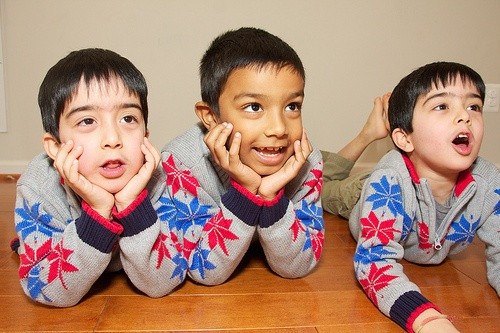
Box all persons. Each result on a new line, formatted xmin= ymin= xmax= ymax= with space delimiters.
xmin=8 ymin=48 xmax=190 ymax=306
xmin=146 ymin=27 xmax=325 ymax=286
xmin=311 ymin=60 xmax=500 ymax=333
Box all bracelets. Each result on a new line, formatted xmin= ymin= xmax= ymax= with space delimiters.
xmin=409 ymin=314 xmax=453 ymax=333
